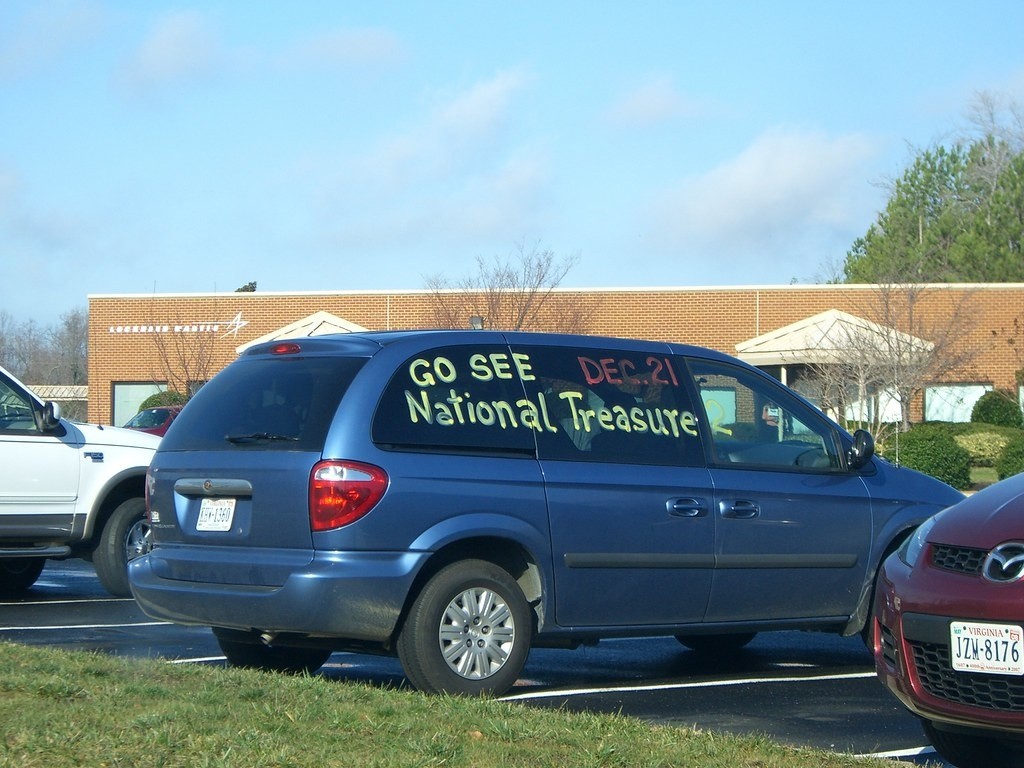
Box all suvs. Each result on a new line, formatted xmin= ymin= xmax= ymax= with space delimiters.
xmin=128 ymin=328 xmax=971 ymax=703
xmin=125 ymin=405 xmax=183 ymax=439
xmin=0 ymin=366 xmax=165 ymax=598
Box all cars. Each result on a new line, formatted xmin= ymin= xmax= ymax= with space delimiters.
xmin=870 ymin=471 xmax=1024 ymax=768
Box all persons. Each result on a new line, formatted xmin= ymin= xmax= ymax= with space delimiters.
xmin=763 ymin=400 xmax=780 ymax=427
